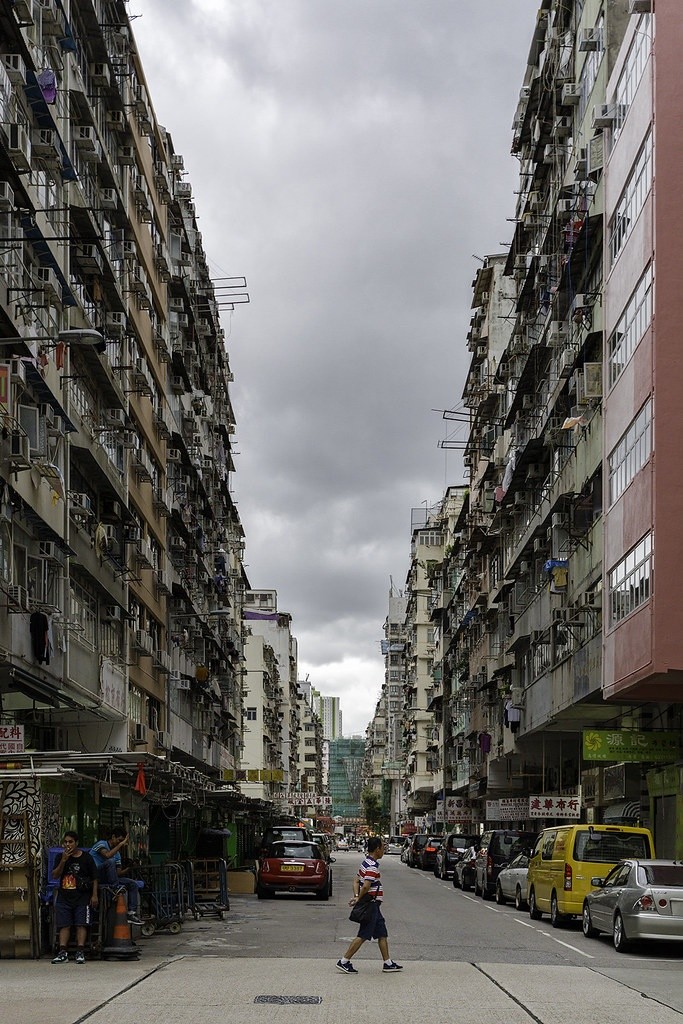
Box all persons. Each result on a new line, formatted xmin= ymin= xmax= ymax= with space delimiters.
xmin=51 ymin=831 xmax=100 ymax=963
xmin=359 ymin=836 xmax=366 ymax=853
xmin=335 ymin=837 xmax=404 ymax=973
xmin=89 ymin=827 xmax=145 ymax=924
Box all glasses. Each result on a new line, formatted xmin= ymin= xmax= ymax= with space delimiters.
xmin=64 ymin=839 xmax=74 ymax=843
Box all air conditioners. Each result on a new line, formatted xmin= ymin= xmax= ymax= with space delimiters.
xmin=515 ymin=255 xmax=526 ymax=278
xmin=509 ymin=334 xmax=522 ymax=351
xmin=551 ymin=513 xmax=570 ymax=528
xmin=517 ymin=394 xmax=536 ymax=419
xmin=545 ymin=320 xmax=570 ymax=348
xmin=434 ymin=291 xmax=510 ymax=685
xmin=526 ymin=463 xmax=544 ymax=477
xmin=534 ymin=537 xmax=550 ymax=552
xmin=563 ymin=608 xmax=585 ymax=625
xmin=512 ymin=686 xmax=526 ymax=705
xmin=0 ymin=0 xmax=302 ymax=749
xmin=549 ymin=417 xmax=562 ymax=431
xmin=514 ymin=491 xmax=528 ymax=504
xmin=511 ymin=9 xmax=614 ymax=231
xmin=521 ymin=561 xmax=533 ymax=571
xmin=578 ymin=592 xmax=600 ymax=607
xmin=568 ymin=368 xmax=585 ymax=394
xmin=530 ymin=628 xmax=550 ymax=643
xmin=551 ymin=607 xmax=563 ymax=622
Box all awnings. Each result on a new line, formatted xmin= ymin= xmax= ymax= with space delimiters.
xmin=604 ymin=801 xmax=640 ymax=818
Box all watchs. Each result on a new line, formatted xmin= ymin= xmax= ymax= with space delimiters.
xmin=354 ymin=893 xmax=359 ymax=897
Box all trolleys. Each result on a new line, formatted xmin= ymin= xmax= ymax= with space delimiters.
xmin=49 ymin=888 xmax=106 ymax=959
xmin=141 ymin=857 xmax=230 ymax=936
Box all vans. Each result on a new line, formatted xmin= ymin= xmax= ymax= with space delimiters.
xmin=521 ymin=824 xmax=655 ymax=927
xmin=473 ymin=830 xmax=539 ymax=900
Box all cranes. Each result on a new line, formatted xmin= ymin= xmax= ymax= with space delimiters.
xmin=343 ymin=731 xmax=362 ymax=739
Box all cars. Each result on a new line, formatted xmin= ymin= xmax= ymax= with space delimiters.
xmin=381 ymin=834 xmax=446 ymax=871
xmin=496 ymin=849 xmax=546 ymax=910
xmin=256 ymin=826 xmax=349 ymax=900
xmin=453 ymin=846 xmax=476 ymax=891
xmin=581 ymin=858 xmax=683 ymax=952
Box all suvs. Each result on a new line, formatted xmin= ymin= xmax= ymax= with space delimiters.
xmin=433 ymin=834 xmax=482 ymax=880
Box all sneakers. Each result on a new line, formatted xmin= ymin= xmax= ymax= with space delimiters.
xmin=51 ymin=947 xmax=69 ymax=963
xmin=75 ymin=949 xmax=85 ymax=964
xmin=335 ymin=960 xmax=358 ymax=974
xmin=382 ymin=960 xmax=403 ymax=972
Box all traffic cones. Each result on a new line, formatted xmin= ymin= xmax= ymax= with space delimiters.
xmin=100 ymin=893 xmax=143 ymax=963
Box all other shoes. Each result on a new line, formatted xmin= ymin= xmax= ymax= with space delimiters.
xmin=113 ymin=886 xmax=122 ymax=896
xmin=127 ymin=914 xmax=145 ymax=925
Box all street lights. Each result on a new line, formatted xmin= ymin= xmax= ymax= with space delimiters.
xmin=381 ymin=766 xmax=401 ymax=836
xmin=266 ymin=740 xmax=293 ymax=797
xmin=406 ymin=706 xmax=446 ymax=835
xmin=166 ymin=610 xmax=230 ymax=772
xmin=354 ymin=822 xmax=357 ymax=847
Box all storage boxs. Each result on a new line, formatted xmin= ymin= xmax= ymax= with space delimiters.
xmin=227 ymin=872 xmax=256 ymax=894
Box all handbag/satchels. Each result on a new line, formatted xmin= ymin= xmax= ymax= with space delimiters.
xmin=74 ymin=875 xmax=94 ymax=893
xmin=348 ymin=896 xmax=375 ymax=923
